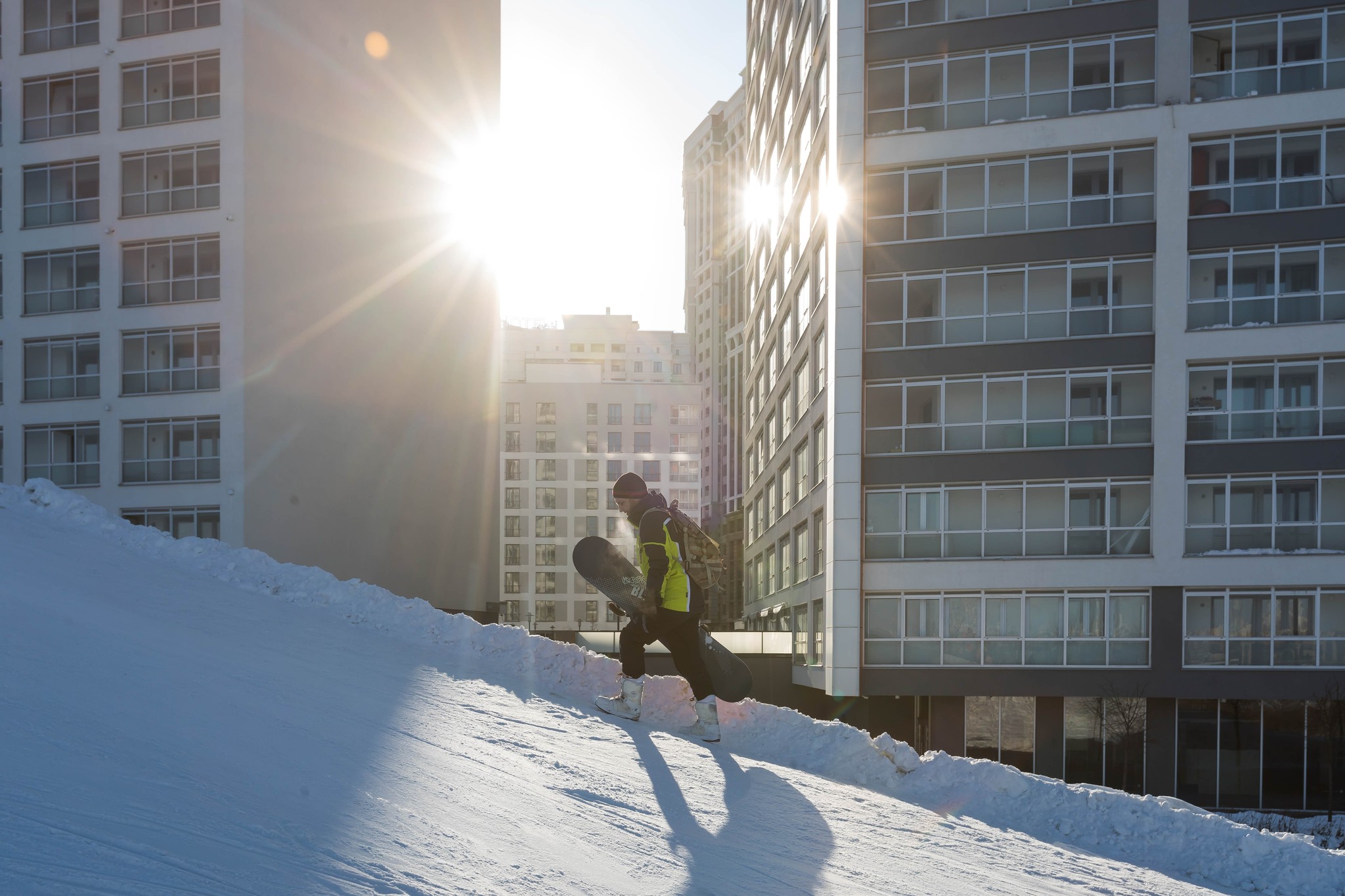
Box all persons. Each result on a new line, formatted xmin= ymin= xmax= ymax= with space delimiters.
xmin=595 ymin=472 xmax=722 ymax=743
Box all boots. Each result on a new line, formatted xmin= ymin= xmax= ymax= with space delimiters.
xmin=678 ymin=694 xmax=721 ymax=743
xmin=595 ymin=672 xmax=646 ymax=720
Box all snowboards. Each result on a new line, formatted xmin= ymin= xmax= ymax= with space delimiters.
xmin=571 ymin=536 xmax=754 ymax=703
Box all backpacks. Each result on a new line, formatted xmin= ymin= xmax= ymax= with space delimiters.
xmin=637 ymin=499 xmax=729 ymax=592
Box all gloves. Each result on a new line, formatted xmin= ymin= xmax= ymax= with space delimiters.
xmin=639 ymin=589 xmax=662 ymax=617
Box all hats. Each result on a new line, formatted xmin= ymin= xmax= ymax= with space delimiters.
xmin=612 ymin=472 xmax=649 ymax=498
